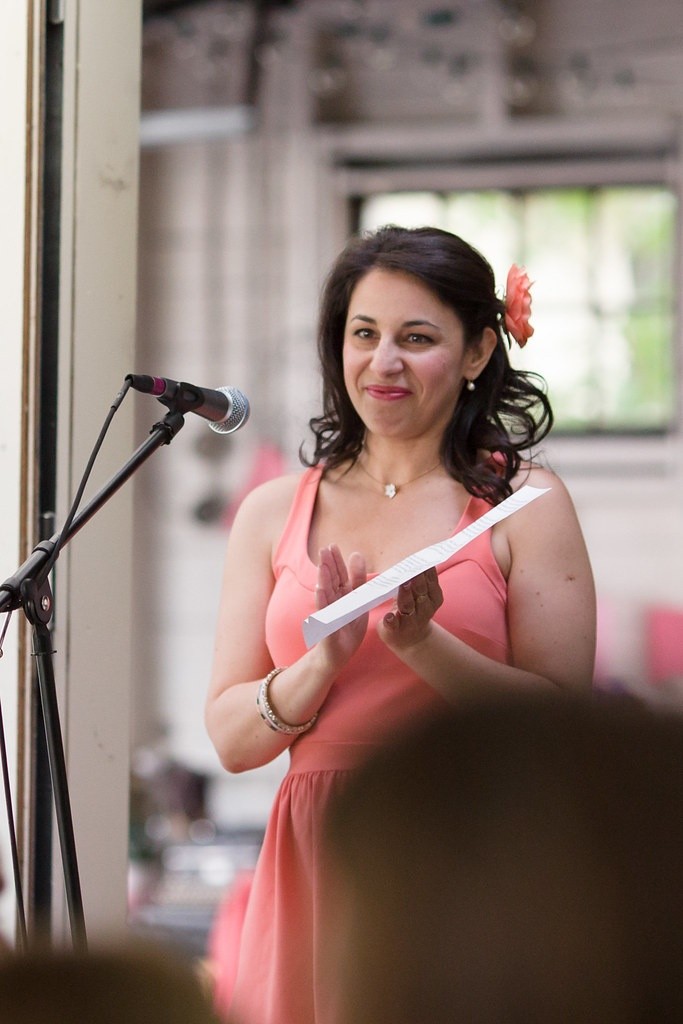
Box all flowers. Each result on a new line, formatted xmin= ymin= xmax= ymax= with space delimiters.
xmin=496 ymin=263 xmax=535 ymax=350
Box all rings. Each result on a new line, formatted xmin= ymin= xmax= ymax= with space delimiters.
xmin=416 ymin=595 xmax=428 ymax=604
xmin=397 ymin=609 xmax=415 ymax=616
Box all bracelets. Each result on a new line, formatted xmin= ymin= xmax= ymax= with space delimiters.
xmin=256 ymin=667 xmax=318 ymax=735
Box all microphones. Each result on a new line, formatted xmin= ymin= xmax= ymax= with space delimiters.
xmin=125 ymin=375 xmax=250 ymax=435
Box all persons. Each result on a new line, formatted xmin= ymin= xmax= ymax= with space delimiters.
xmin=309 ymin=670 xmax=683 ymax=1024
xmin=201 ymin=226 xmax=597 ymax=1024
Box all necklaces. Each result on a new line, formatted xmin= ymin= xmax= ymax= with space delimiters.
xmin=358 ymin=458 xmax=440 ymax=498
